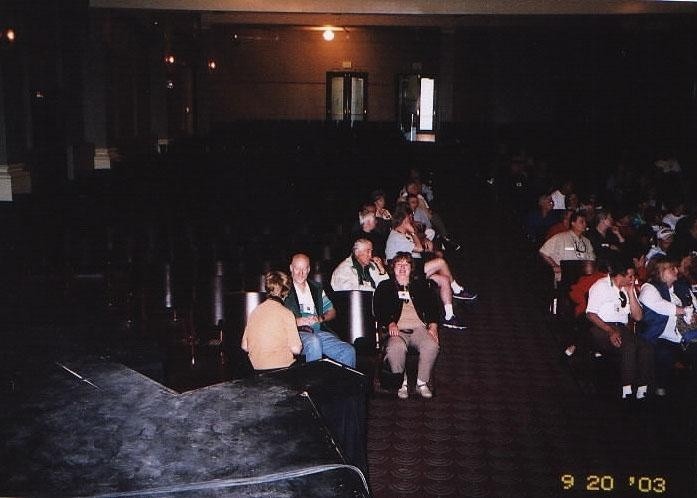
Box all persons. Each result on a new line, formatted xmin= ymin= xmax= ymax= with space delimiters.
xmin=519 ymin=191 xmax=697 ymax=408
xmin=240 ymin=269 xmax=305 ymax=373
xmin=284 ymin=254 xmax=358 ymax=369
xmin=328 ymin=168 xmax=478 ymax=400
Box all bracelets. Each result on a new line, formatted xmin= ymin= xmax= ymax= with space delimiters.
xmin=319 ymin=314 xmax=325 ymax=323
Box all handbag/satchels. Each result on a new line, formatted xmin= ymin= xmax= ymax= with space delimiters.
xmin=676 ymin=313 xmax=696 ymax=353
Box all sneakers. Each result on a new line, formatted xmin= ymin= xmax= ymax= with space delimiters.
xmin=442 ymin=316 xmax=468 ymax=330
xmin=452 ymin=288 xmax=477 ymax=300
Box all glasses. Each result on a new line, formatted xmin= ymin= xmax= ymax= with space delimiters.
xmin=576 ymin=240 xmax=586 ymax=253
xmin=620 ymin=291 xmax=626 ymax=308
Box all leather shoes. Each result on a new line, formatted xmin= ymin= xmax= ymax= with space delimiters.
xmin=398 ymin=385 xmax=408 ymax=399
xmin=415 ymin=384 xmax=432 ymax=399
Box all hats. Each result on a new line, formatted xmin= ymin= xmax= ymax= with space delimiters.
xmin=656 ymin=228 xmax=675 ymax=241
